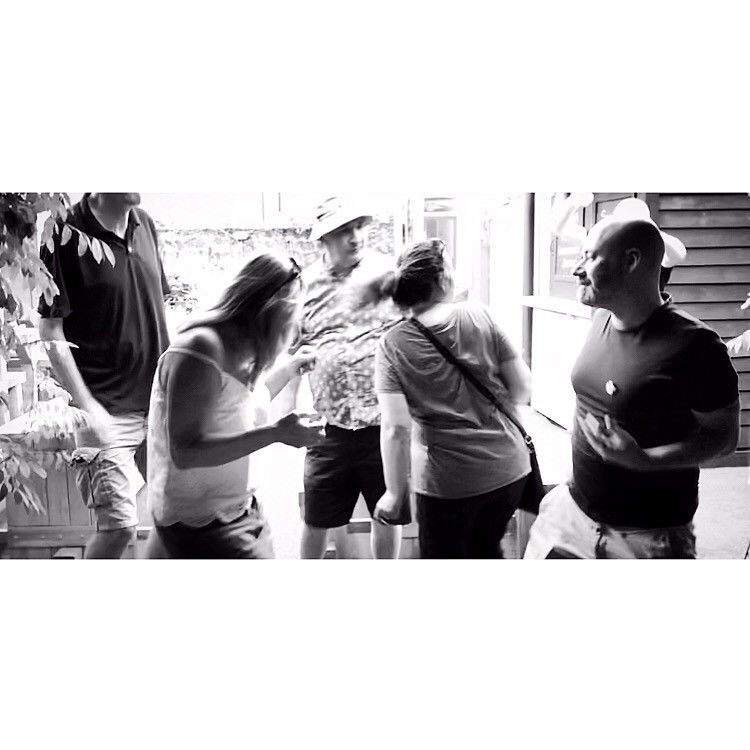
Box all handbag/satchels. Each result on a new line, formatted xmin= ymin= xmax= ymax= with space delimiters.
xmin=516 ymin=436 xmax=545 ymax=516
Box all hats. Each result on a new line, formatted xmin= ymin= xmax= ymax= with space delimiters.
xmin=308 ymin=196 xmax=374 ymax=241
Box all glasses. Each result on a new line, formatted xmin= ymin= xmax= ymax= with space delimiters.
xmin=430 ymin=238 xmax=448 ymax=258
xmin=259 ymin=258 xmax=302 ymax=308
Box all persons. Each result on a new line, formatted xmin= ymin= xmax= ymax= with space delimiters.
xmin=38 ymin=192 xmax=171 ymax=558
xmin=147 ymin=253 xmax=327 ymax=560
xmin=600 ymin=198 xmax=686 ymax=296
xmin=520 ymin=215 xmax=741 ymax=559
xmin=272 ymin=196 xmax=412 ymax=559
xmin=371 ymin=237 xmax=533 ymax=560
xmin=725 ymin=292 xmax=750 ymax=353
